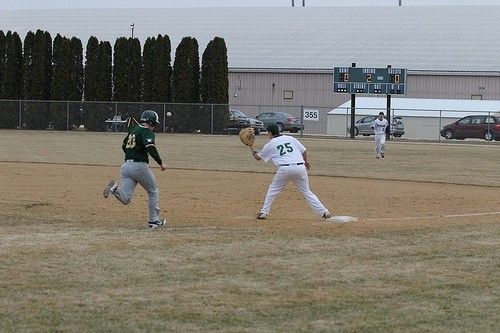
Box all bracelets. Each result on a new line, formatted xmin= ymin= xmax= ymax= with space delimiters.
xmin=252 ymin=151 xmax=258 ymax=156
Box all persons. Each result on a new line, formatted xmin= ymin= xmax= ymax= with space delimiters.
xmin=371 ymin=112 xmax=389 ymax=159
xmin=249 ymin=123 xmax=331 ymax=220
xmin=103 ymin=110 xmax=167 ymax=229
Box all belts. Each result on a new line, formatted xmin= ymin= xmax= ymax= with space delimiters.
xmin=280 ymin=163 xmax=303 ymax=166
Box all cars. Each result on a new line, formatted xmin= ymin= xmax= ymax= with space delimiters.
xmin=254 ymin=111 xmax=305 ymax=133
xmin=228 ymin=109 xmax=265 ymax=135
xmin=440 ymin=115 xmax=500 ymax=142
xmin=347 ymin=114 xmax=405 ymax=137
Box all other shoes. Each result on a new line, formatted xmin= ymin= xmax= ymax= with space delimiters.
xmin=323 ymin=212 xmax=331 ymax=220
xmin=148 ymin=219 xmax=166 ymax=230
xmin=256 ymin=212 xmax=267 ymax=220
xmin=382 ymin=156 xmax=384 ymax=158
xmin=376 ymin=157 xmax=380 ymax=159
xmin=103 ymin=180 xmax=119 ymax=199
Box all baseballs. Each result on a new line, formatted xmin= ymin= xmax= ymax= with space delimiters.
xmin=166 ymin=112 xmax=171 ymax=117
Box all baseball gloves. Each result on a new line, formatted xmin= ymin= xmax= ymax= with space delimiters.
xmin=239 ymin=127 xmax=256 ymax=147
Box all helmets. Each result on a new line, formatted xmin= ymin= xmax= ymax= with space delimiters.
xmin=264 ymin=123 xmax=279 ymax=132
xmin=140 ymin=110 xmax=160 ymax=127
